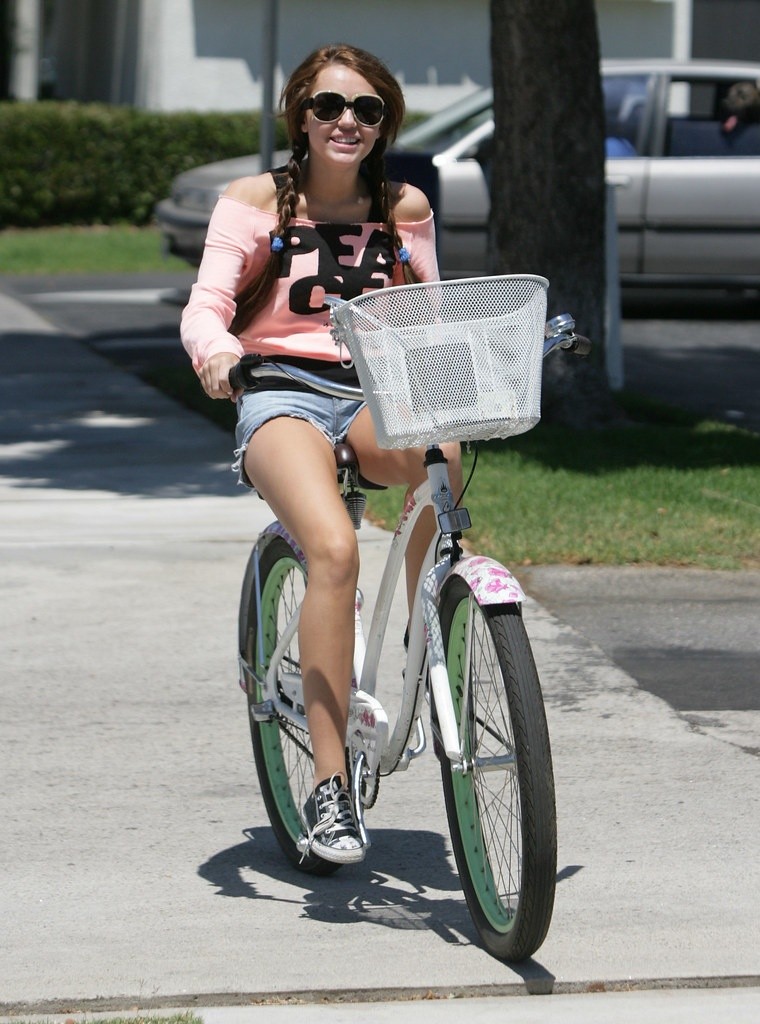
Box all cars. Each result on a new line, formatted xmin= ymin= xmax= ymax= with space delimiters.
xmin=153 ymin=57 xmax=759 ymax=287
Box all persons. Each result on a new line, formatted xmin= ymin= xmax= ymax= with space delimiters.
xmin=605 ymin=87 xmax=638 ymax=157
xmin=715 ymin=81 xmax=760 ymax=122
xmin=179 ymin=45 xmax=464 ymax=865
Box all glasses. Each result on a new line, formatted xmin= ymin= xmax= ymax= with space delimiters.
xmin=299 ymin=89 xmax=387 ymax=127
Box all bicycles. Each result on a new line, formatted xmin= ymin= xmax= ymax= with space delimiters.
xmin=226 ymin=309 xmax=595 ymax=960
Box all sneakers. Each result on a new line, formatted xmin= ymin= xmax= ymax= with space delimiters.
xmin=303 ymin=776 xmax=366 ymax=863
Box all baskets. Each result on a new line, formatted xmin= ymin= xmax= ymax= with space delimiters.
xmin=332 ymin=272 xmax=549 ymax=450
